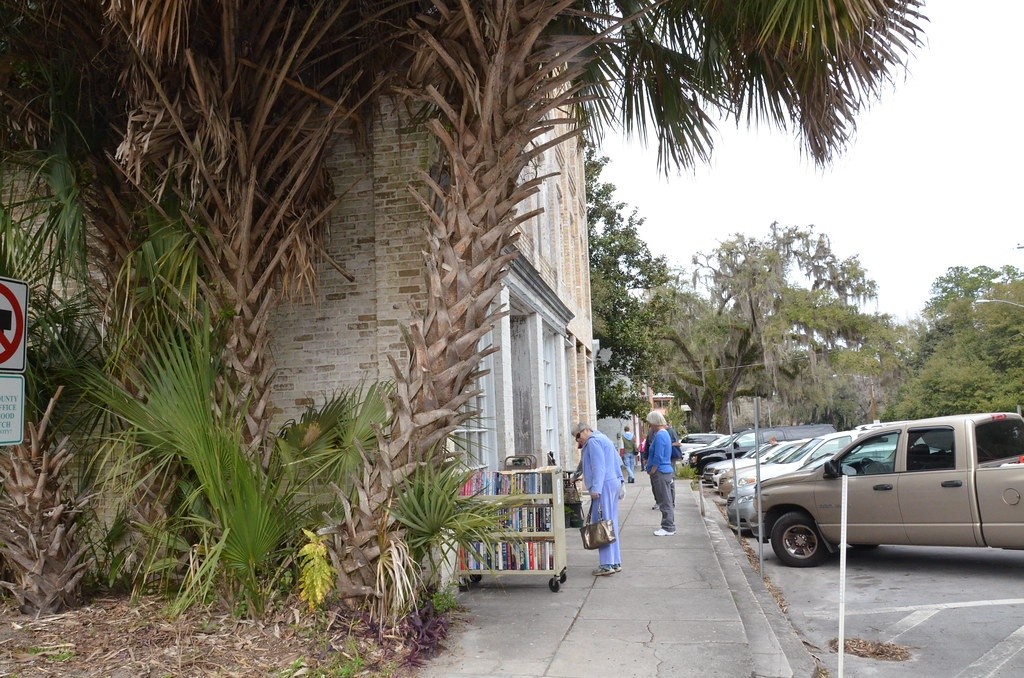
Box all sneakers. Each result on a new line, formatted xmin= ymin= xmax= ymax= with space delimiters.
xmin=591 ymin=564 xmax=615 ymax=576
xmin=613 ymin=564 xmax=622 ymax=572
xmin=653 ymin=528 xmax=676 ymax=535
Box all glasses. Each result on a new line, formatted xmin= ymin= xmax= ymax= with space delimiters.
xmin=575 ymin=428 xmax=594 ymax=442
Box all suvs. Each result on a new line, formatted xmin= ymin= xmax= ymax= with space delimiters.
xmin=689 ymin=422 xmax=837 ymax=477
xmin=678 ymin=433 xmax=725 ymax=453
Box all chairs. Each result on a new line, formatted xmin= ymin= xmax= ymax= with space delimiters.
xmin=908 ymin=443 xmax=931 ymax=471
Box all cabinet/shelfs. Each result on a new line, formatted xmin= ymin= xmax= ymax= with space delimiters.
xmin=459 ymin=465 xmax=567 ymax=593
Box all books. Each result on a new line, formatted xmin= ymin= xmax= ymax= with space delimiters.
xmin=457 ymin=473 xmax=554 ymax=570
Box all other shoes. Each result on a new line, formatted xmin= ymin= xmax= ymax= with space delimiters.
xmin=630 ymin=478 xmax=635 ymax=483
xmin=651 ymin=504 xmax=660 ymax=511
xmin=628 ymin=479 xmax=631 ymax=483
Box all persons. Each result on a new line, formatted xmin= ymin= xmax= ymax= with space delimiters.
xmin=640 ymin=411 xmax=682 ymax=535
xmin=571 ymin=424 xmax=625 ymax=576
xmin=623 ymin=427 xmax=638 ymax=483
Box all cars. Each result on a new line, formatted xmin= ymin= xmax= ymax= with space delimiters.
xmin=683 ymin=434 xmax=755 ymax=467
xmin=704 ymin=422 xmax=937 ymax=537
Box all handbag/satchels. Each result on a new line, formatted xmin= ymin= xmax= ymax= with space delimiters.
xmin=633 ymin=448 xmax=638 ymax=455
xmin=580 ymin=494 xmax=616 ymax=550
xmin=667 ymin=430 xmax=683 ymax=459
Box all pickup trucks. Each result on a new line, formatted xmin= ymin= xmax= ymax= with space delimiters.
xmin=749 ymin=411 xmax=1023 ymax=568
